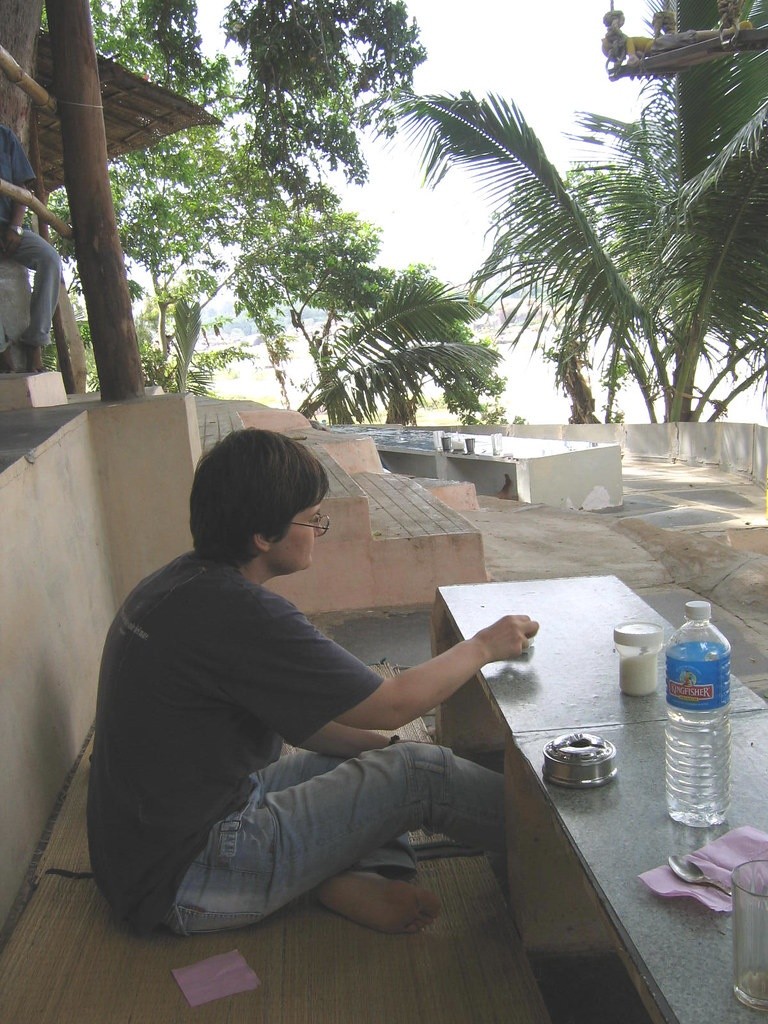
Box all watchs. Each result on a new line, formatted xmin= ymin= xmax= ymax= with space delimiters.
xmin=9 ymin=224 xmax=24 ymax=236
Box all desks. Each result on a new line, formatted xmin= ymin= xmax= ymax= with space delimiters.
xmin=428 ymin=570 xmax=768 ymax=1024
xmin=329 ymin=425 xmax=630 ymax=507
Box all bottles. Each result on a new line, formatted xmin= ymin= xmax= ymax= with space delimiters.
xmin=662 ymin=600 xmax=733 ymax=829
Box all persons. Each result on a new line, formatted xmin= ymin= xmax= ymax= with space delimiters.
xmin=0 ymin=125 xmax=62 ymax=374
xmin=85 ymin=427 xmax=539 ymax=937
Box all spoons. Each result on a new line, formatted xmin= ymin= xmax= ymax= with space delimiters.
xmin=667 ymin=855 xmax=732 ymax=897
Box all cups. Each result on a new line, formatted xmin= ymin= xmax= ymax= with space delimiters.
xmin=465 ymin=438 xmax=475 ymax=452
xmin=731 ymin=860 xmax=768 ymax=1011
xmin=491 ymin=433 xmax=503 ymax=455
xmin=614 ymin=620 xmax=664 ymax=697
xmin=432 ymin=430 xmax=464 ymax=453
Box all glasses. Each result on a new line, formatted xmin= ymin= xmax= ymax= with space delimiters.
xmin=288 ymin=513 xmax=330 ymax=537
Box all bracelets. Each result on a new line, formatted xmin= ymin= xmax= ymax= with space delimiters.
xmin=389 ymin=734 xmax=400 ymax=744
